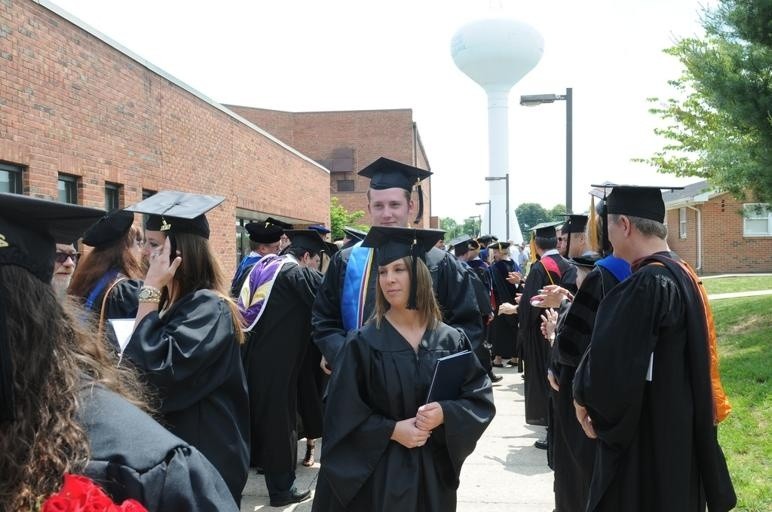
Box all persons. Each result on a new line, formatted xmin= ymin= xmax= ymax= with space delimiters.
xmin=311 ymin=226 xmax=497 ymax=512
xmin=522 ymin=241 xmax=532 ymax=263
xmin=343 ymin=226 xmax=367 ymax=248
xmin=119 ymin=189 xmax=250 ymax=507
xmin=572 ymin=182 xmax=739 ymax=512
xmin=517 ymin=245 xmax=528 ymax=279
xmin=501 ymin=243 xmax=511 ymax=259
xmin=469 ymin=239 xmax=492 ymax=296
xmin=520 ymin=220 xmax=572 ymax=450
xmin=548 ymin=180 xmax=630 ymax=512
xmin=312 ymin=155 xmax=484 ymax=375
xmin=530 ymin=212 xmax=589 ymax=471
xmin=450 ymin=235 xmax=503 ymax=383
xmin=237 ymin=228 xmax=333 ymax=507
xmin=228 ymin=223 xmax=293 ymax=302
xmin=51 ymin=240 xmax=121 ymax=384
xmin=66 ymin=209 xmax=143 ymax=319
xmin=0 ymin=189 xmax=241 ymax=512
xmin=488 ymin=242 xmax=519 ymax=368
xmin=540 ymin=250 xmax=601 ymax=495
xmin=297 ymin=241 xmax=338 ymax=466
xmin=477 ymin=234 xmax=491 ymax=263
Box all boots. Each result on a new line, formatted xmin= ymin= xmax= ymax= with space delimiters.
xmin=301 ymin=446 xmax=315 ymax=466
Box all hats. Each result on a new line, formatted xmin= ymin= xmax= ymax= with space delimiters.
xmin=357 ymin=156 xmax=433 ymax=191
xmin=359 ymin=225 xmax=447 ymax=266
xmin=529 ymin=221 xmax=565 ymax=239
xmin=591 ymin=184 xmax=685 ymax=224
xmin=589 ymin=181 xmax=613 ymax=215
xmin=489 ymin=242 xmax=510 ymax=251
xmin=282 ymin=227 xmax=330 ymax=256
xmin=556 ymin=215 xmax=589 ymax=233
xmin=245 ymin=218 xmax=285 ymax=245
xmin=450 ymin=234 xmax=472 ymax=258
xmin=123 ymin=188 xmax=226 ymax=234
xmin=476 ymin=235 xmax=498 ymax=244
xmin=308 ymin=225 xmax=331 ymax=232
xmin=1 ymin=191 xmax=110 ymax=286
xmin=340 ymin=226 xmax=368 ymax=242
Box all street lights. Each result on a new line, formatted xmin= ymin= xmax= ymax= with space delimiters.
xmin=520 ymin=87 xmax=574 ymax=223
xmin=476 ymin=201 xmax=492 ymax=234
xmin=484 ymin=173 xmax=509 ymax=242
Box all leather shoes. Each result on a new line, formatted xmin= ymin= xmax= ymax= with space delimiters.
xmin=490 ymin=375 xmax=503 ymax=383
xmin=535 ymin=439 xmax=548 ymax=447
xmin=271 ymin=489 xmax=311 ymax=507
xmin=507 ymin=360 xmax=518 ymax=366
xmin=492 ymin=363 xmax=503 ymax=367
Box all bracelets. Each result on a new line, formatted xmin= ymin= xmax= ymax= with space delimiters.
xmin=136 ymin=285 xmax=161 ymax=305
